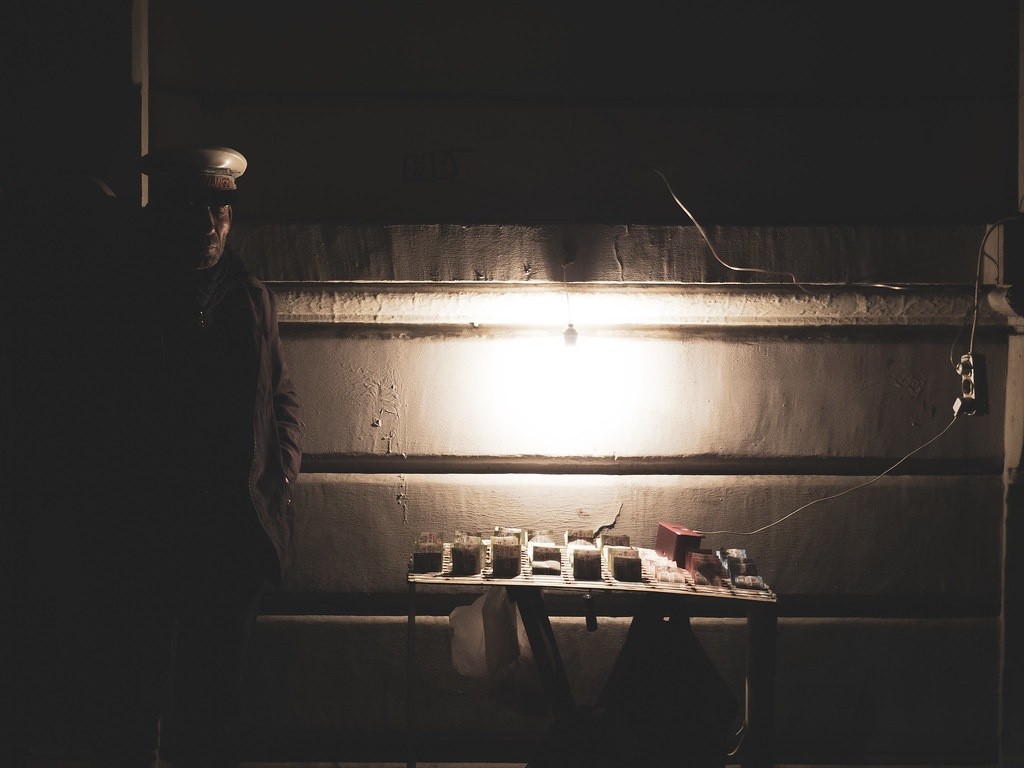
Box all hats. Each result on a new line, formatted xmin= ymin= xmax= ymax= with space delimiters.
xmin=138 ymin=146 xmax=247 ymax=205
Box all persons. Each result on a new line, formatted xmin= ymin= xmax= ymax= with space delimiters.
xmin=67 ymin=146 xmax=301 ymax=768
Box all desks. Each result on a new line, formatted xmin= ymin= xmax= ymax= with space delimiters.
xmin=405 ymin=539 xmax=781 ymax=768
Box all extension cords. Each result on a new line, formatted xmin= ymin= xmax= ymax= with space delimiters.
xmin=961 ymin=352 xmax=988 ymax=417
